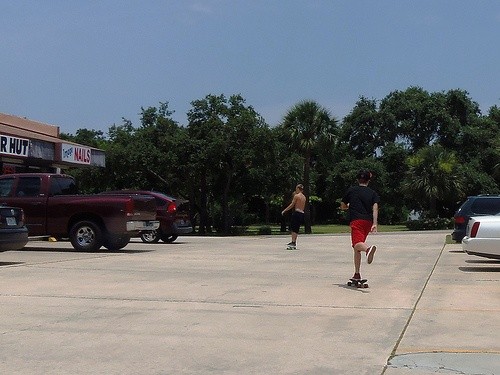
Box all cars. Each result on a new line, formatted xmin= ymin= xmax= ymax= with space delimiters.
xmin=407 ymin=209 xmax=421 ymax=222
xmin=0 ymin=203 xmax=29 ymax=253
xmin=461 ymin=211 xmax=500 ymax=260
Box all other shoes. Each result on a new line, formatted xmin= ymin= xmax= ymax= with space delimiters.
xmin=352 ymin=273 xmax=360 ymax=281
xmin=288 ymin=242 xmax=296 ymax=247
xmin=366 ymin=246 xmax=376 ymax=264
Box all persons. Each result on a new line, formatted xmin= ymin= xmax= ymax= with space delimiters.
xmin=340 ymin=169 xmax=379 ymax=278
xmin=282 ymin=185 xmax=306 ymax=246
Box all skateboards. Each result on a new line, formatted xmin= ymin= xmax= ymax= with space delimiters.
xmin=347 ymin=277 xmax=369 ymax=289
xmin=286 ymin=242 xmax=296 ymax=250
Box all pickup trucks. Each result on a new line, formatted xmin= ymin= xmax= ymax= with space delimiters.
xmin=0 ymin=173 xmax=160 ymax=252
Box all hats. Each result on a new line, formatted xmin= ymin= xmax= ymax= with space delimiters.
xmin=356 ymin=169 xmax=372 ymax=180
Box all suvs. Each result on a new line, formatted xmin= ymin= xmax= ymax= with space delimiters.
xmin=451 ymin=194 xmax=500 ymax=244
xmin=97 ymin=190 xmax=193 ymax=243
